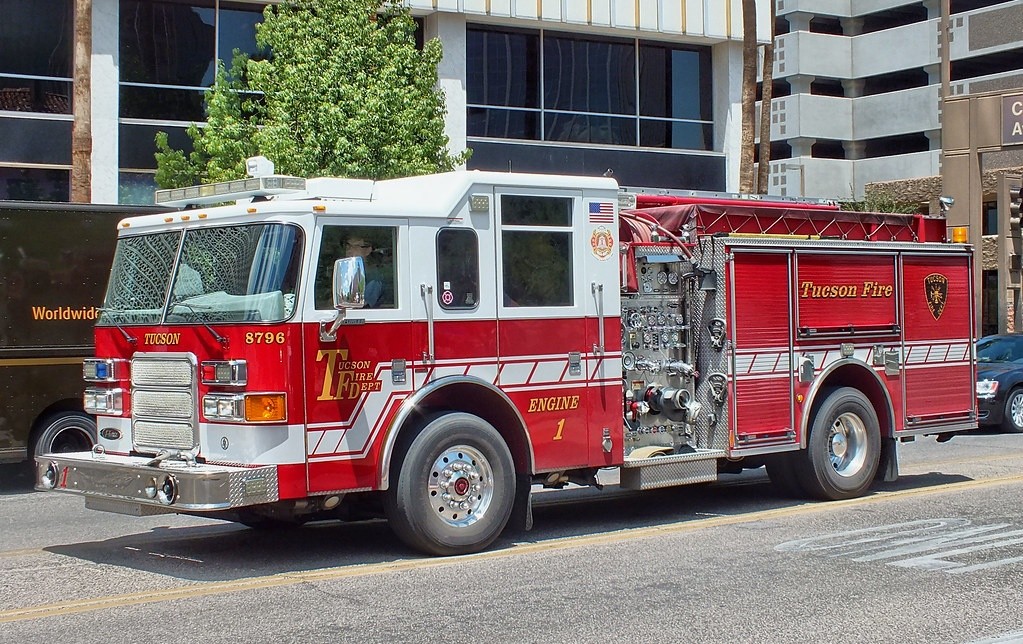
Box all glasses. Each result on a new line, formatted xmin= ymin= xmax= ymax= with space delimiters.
xmin=342 ymin=241 xmax=367 ymax=251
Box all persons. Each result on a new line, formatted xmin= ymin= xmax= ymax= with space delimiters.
xmin=322 ymin=228 xmax=386 ymax=308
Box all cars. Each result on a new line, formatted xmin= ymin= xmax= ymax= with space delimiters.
xmin=969 ymin=333 xmax=1023 ymax=433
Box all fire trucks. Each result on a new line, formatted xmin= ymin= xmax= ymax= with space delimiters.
xmin=29 ymin=153 xmax=989 ymax=557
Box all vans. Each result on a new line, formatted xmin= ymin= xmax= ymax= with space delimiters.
xmin=0 ymin=197 xmax=216 ymax=489
